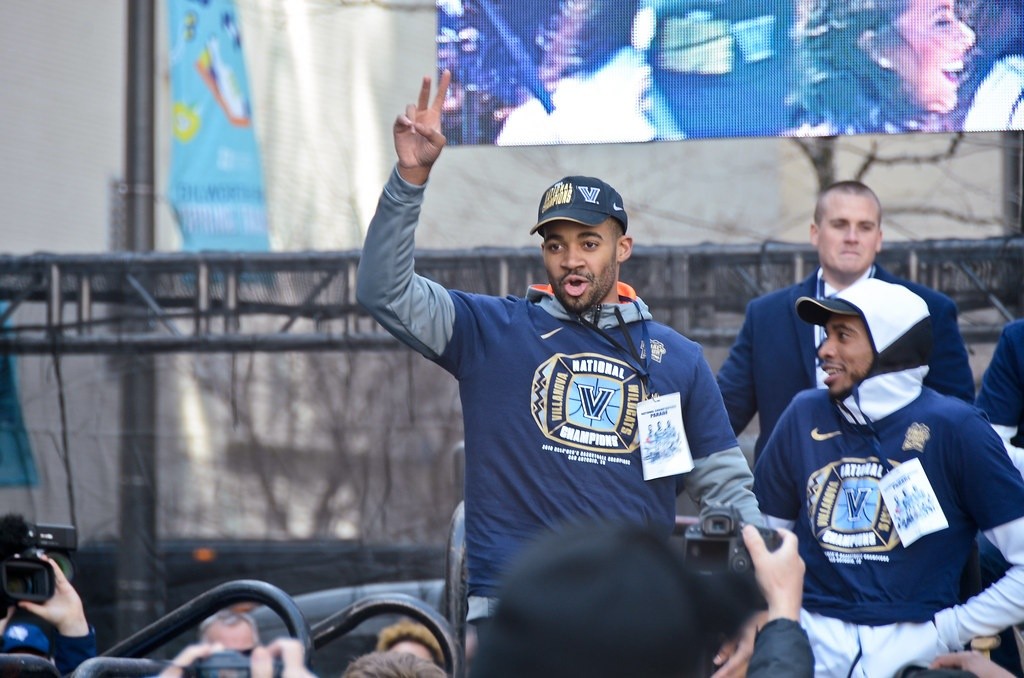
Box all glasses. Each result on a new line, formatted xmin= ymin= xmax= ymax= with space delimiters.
xmin=242 ymin=647 xmax=256 ymax=656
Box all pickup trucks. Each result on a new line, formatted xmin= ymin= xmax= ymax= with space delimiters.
xmin=0 ymin=498 xmax=466 ymax=677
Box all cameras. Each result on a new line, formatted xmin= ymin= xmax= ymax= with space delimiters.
xmin=682 ymin=502 xmax=783 ymax=579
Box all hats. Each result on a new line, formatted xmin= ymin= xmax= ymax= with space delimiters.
xmin=1 ymin=623 xmax=50 ymax=660
xmin=795 ymin=296 xmax=860 ymax=326
xmin=530 ymin=176 xmax=627 ymax=235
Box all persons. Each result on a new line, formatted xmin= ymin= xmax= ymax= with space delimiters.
xmin=717 ymin=180 xmax=973 ymax=471
xmin=353 ymin=67 xmax=771 ymax=642
xmin=0 ymin=523 xmax=1024 ymax=678
xmin=752 ymin=277 xmax=1024 ymax=678
xmin=437 ymin=0 xmax=1024 ymax=145
xmin=974 ymin=318 xmax=1024 ymax=481
